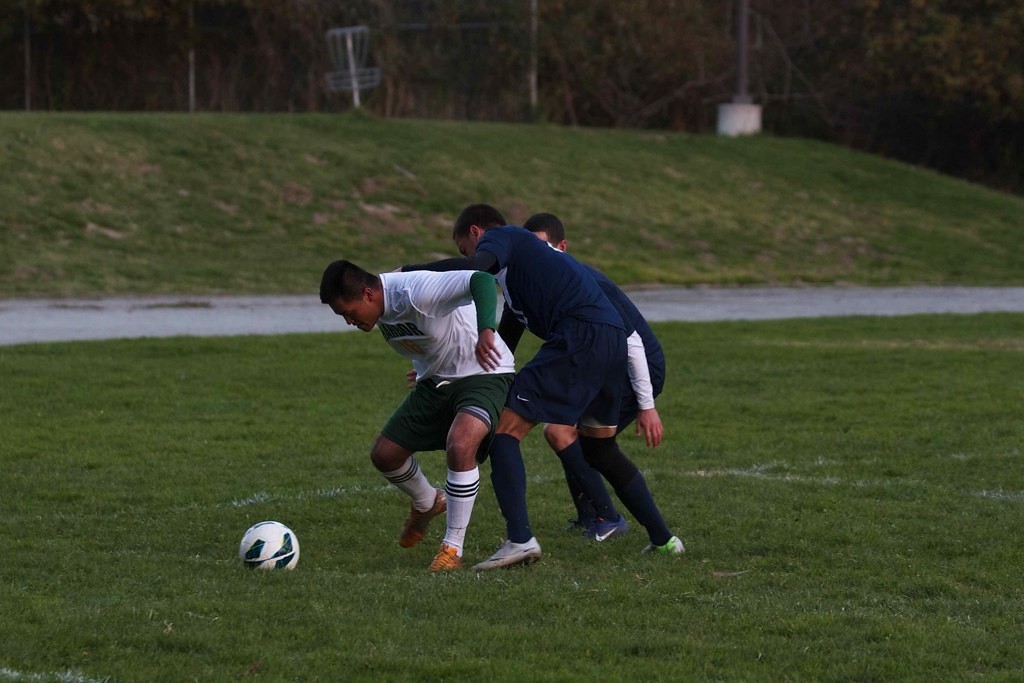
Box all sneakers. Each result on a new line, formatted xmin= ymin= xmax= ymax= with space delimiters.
xmin=582 ymin=514 xmax=628 ymax=543
xmin=641 ymin=535 xmax=685 ymax=556
xmin=564 ymin=516 xmax=606 ymax=532
xmin=429 ymin=542 xmax=462 ymax=573
xmin=470 ymin=536 xmax=542 ymax=571
xmin=398 ymin=488 xmax=447 ymax=548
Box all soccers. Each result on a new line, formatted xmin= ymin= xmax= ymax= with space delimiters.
xmin=237 ymin=519 xmax=301 ymax=572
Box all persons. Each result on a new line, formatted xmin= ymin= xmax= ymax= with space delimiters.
xmin=319 ymin=259 xmax=517 ymax=571
xmin=390 ymin=203 xmax=685 ymax=571
xmin=497 ymin=212 xmax=666 ymax=542
